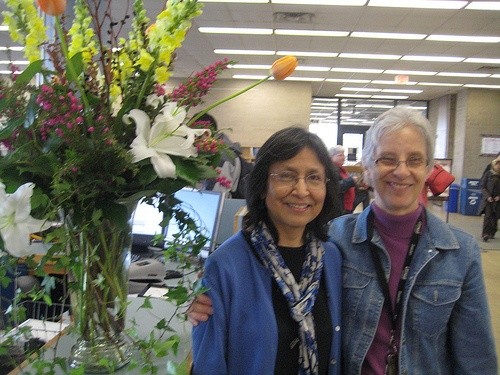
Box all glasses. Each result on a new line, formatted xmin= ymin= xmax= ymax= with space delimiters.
xmin=373 ymin=154 xmax=429 ymax=170
xmin=268 ymin=171 xmax=331 ymax=189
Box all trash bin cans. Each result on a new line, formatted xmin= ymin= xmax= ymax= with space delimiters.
xmin=462 ymin=177 xmax=483 ymax=216
xmin=449 ymin=184 xmax=459 ymax=213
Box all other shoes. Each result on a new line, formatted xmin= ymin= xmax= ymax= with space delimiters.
xmin=488 ymin=234 xmax=494 ymax=239
xmin=483 ymin=234 xmax=489 ymax=241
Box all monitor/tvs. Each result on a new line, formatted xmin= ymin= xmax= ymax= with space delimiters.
xmin=129 ymin=187 xmax=226 ymax=259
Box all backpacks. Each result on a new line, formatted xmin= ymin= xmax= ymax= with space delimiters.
xmin=228 ymin=151 xmax=252 ymax=200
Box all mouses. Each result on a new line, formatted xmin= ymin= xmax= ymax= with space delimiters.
xmin=164 ymin=270 xmax=184 ymax=279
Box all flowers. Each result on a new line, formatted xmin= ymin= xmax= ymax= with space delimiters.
xmin=0 ymin=0 xmax=298 ymax=375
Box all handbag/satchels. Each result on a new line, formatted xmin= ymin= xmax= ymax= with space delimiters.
xmin=424 ymin=165 xmax=455 ymax=197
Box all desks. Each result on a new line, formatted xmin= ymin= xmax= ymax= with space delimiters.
xmin=20 ymin=255 xmax=69 ymax=274
xmin=9 ymin=295 xmax=193 ymax=375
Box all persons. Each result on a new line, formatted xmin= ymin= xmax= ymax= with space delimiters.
xmin=202 ymin=133 xmax=247 ymax=197
xmin=190 ymin=126 xmax=342 ymax=375
xmin=329 ymin=145 xmax=369 ymax=214
xmin=189 ymin=104 xmax=500 ymax=375
xmin=480 ymin=161 xmax=500 ymax=240
xmin=484 ymin=152 xmax=500 ymax=172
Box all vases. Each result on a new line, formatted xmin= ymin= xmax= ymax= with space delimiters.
xmin=56 ymin=198 xmax=133 ymax=372
